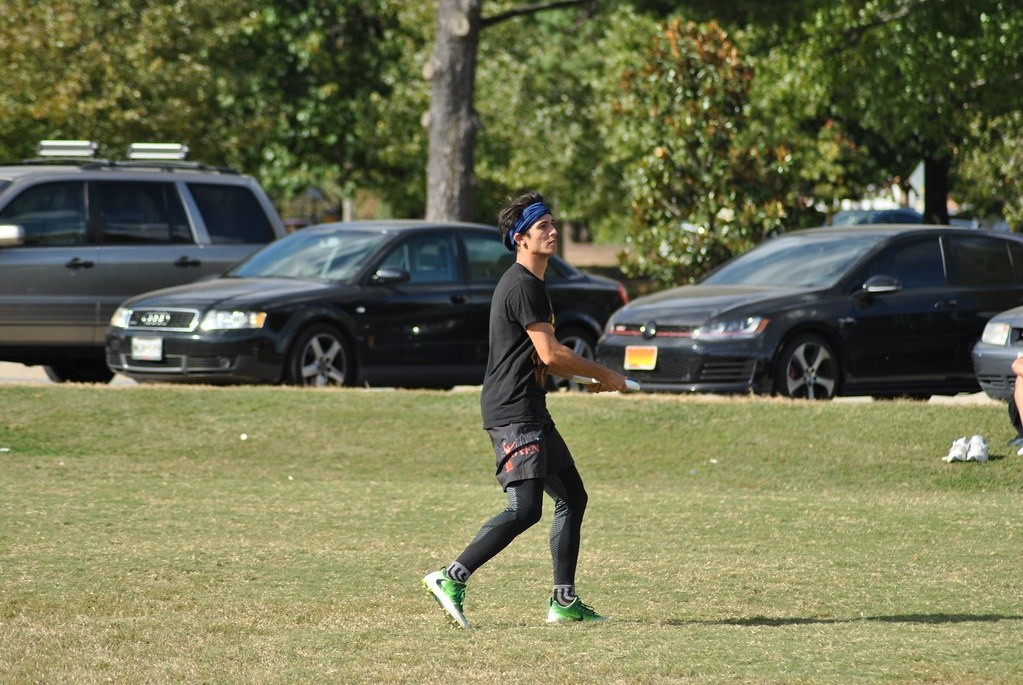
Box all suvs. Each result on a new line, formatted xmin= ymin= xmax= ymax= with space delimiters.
xmin=0 ymin=137 xmax=291 ymax=384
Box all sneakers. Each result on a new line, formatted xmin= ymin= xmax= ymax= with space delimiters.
xmin=547 ymin=595 xmax=606 ymax=624
xmin=421 ymin=566 xmax=470 ymax=630
xmin=965 ymin=435 xmax=988 ymax=462
xmin=946 ymin=436 xmax=971 ymax=462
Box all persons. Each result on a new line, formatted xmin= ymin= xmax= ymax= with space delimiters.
xmin=421 ymin=193 xmax=628 ymax=631
xmin=1007 ymin=352 xmax=1023 ymax=456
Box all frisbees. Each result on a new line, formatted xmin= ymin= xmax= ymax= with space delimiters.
xmin=571 ymin=374 xmax=640 ymax=392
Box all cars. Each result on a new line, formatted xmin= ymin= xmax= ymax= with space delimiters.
xmin=970 ymin=303 xmax=1023 ymax=436
xmin=820 ymin=207 xmax=922 ymax=229
xmin=593 ymin=221 xmax=1023 ymax=401
xmin=949 ymin=197 xmax=1016 ymax=234
xmin=103 ymin=219 xmax=630 ymax=396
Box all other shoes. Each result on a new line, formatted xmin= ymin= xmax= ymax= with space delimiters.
xmin=1017 ymin=448 xmax=1023 ymax=456
